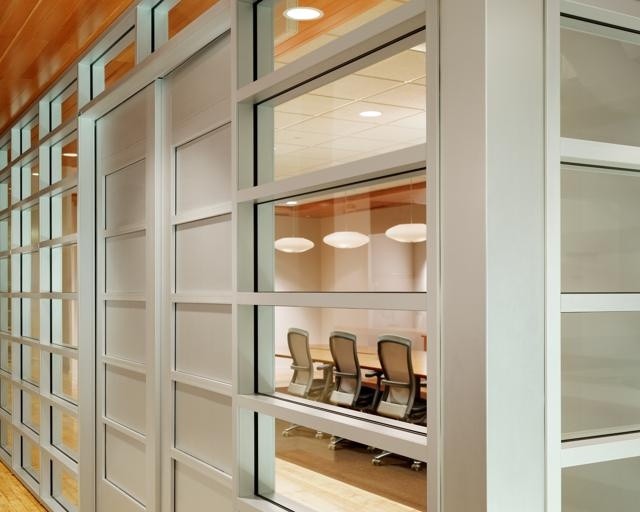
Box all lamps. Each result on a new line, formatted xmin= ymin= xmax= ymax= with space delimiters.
xmin=323 ymin=190 xmax=370 ymax=249
xmin=385 ymin=177 xmax=427 ymax=243
xmin=274 ymin=206 xmax=315 ymax=254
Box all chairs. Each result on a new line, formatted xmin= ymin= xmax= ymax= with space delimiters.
xmin=283 ymin=328 xmax=428 ymax=472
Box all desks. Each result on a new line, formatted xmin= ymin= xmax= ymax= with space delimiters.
xmin=274 ymin=344 xmax=428 ymax=405
